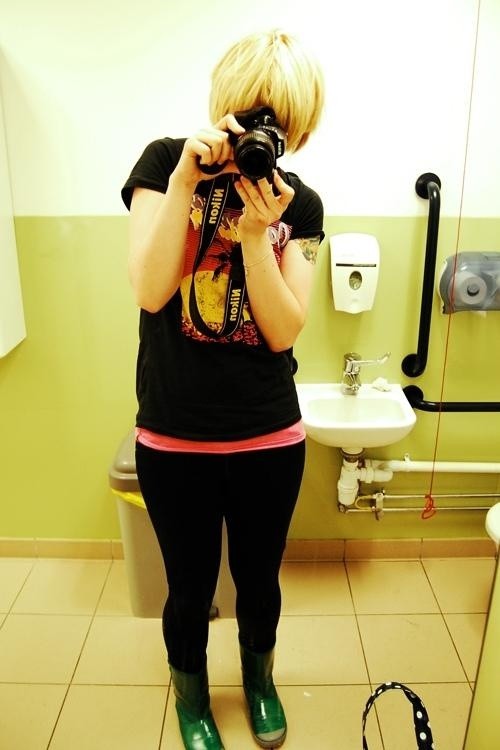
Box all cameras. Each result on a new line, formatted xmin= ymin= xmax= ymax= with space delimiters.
xmin=196 ymin=104 xmax=287 ymax=196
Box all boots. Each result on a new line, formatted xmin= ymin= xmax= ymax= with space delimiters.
xmin=238 ymin=630 xmax=287 ymax=749
xmin=168 ymin=651 xmax=225 ymax=750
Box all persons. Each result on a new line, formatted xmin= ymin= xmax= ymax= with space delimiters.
xmin=121 ymin=30 xmax=325 ymax=750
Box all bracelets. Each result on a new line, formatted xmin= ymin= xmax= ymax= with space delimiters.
xmin=242 ymin=248 xmax=272 ymax=267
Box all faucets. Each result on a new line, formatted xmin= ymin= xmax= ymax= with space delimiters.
xmin=340 ymin=352 xmax=390 ymax=394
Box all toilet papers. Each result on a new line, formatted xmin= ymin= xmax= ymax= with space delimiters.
xmin=455 ymin=271 xmax=488 ymax=317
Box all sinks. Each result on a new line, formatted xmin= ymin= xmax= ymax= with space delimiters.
xmin=296 ymin=384 xmax=416 ymax=447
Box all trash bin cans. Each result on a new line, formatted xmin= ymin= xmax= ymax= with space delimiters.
xmin=105 ymin=429 xmax=170 ymax=618
